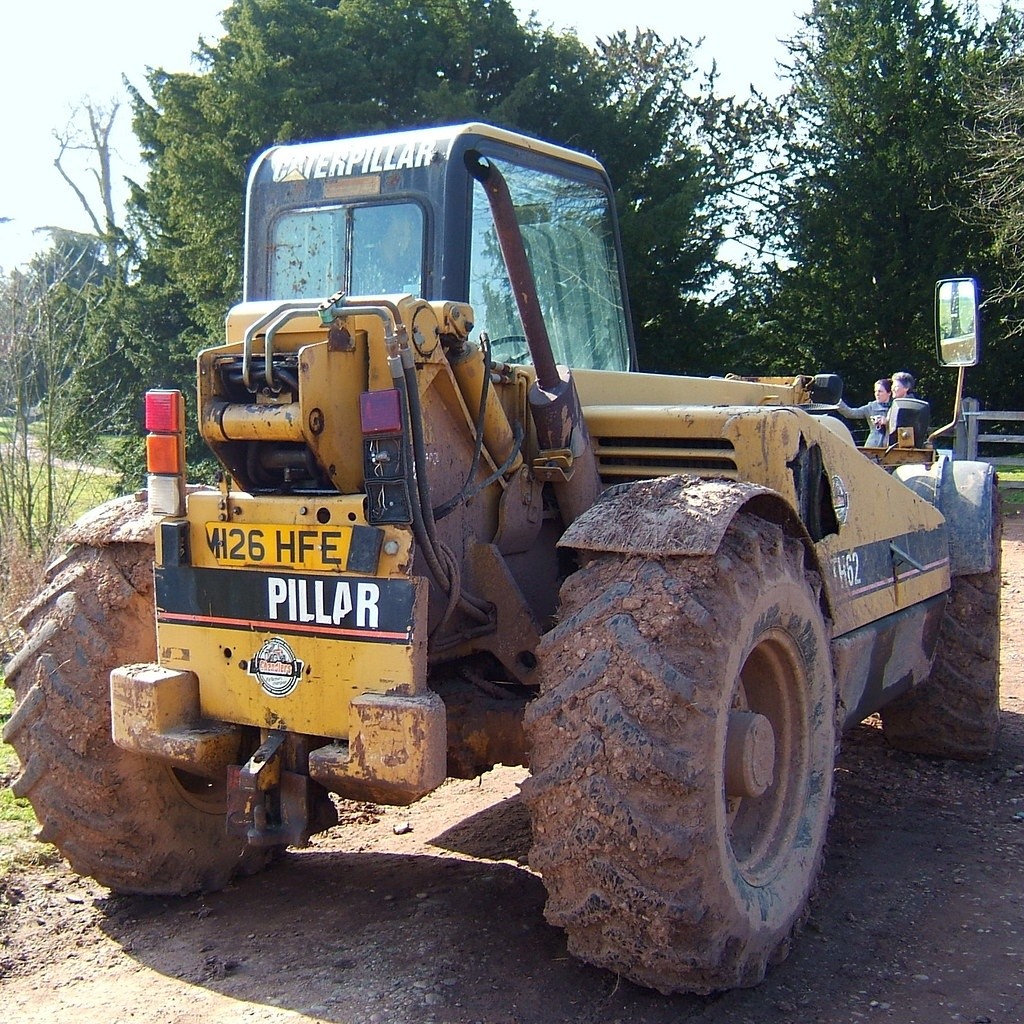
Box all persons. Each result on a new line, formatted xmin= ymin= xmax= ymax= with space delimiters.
xmin=837 ymin=378 xmax=893 ymax=449
xmin=871 ymin=373 xmax=917 ymax=448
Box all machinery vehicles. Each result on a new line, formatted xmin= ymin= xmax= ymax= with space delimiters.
xmin=2 ymin=123 xmax=1002 ymax=995
